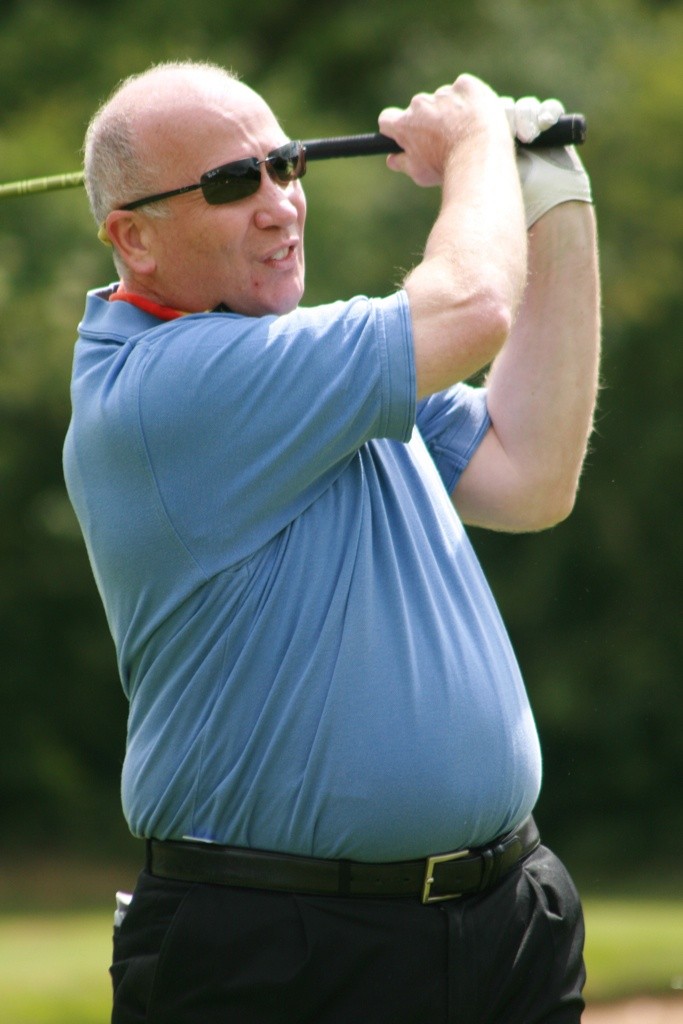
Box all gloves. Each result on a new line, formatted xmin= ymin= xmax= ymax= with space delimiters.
xmin=499 ymin=95 xmax=593 ymax=231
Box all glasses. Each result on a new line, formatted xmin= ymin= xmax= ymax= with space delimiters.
xmin=97 ymin=140 xmax=308 ymax=247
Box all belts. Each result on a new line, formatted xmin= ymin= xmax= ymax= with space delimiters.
xmin=146 ymin=815 xmax=543 ymax=904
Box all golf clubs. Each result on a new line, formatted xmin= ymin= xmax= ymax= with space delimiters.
xmin=1 ymin=109 xmax=589 ymax=202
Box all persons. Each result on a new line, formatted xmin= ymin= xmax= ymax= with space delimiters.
xmin=62 ymin=62 xmax=602 ymax=1023
